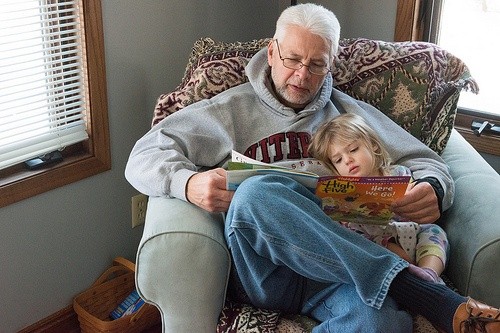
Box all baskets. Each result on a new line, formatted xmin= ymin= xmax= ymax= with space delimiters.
xmin=74 ymin=256 xmax=160 ymax=333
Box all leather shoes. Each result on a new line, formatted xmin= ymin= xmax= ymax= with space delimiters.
xmin=452 ymin=297 xmax=500 ymax=333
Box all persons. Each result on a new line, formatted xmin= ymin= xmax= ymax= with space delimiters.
xmin=312 ymin=113 xmax=448 ymax=278
xmin=125 ymin=4 xmax=500 ymax=333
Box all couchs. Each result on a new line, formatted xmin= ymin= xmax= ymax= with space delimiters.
xmin=134 ymin=38 xmax=500 ymax=333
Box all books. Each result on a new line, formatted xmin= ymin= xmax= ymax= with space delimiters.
xmin=227 ymin=149 xmax=411 ymax=226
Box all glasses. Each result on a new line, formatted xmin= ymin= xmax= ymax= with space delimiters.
xmin=275 ymin=39 xmax=333 ymax=76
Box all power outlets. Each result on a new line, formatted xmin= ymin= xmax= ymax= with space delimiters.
xmin=132 ymin=194 xmax=147 ymax=226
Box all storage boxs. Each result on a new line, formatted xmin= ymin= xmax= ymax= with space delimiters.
xmin=114 ymin=293 xmax=141 ymax=320
